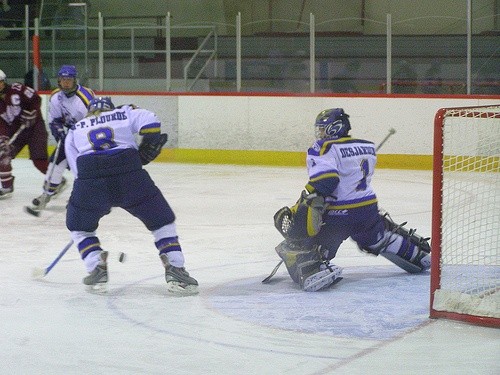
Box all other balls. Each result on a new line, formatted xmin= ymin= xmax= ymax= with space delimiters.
xmin=120 ymin=253 xmax=124 ymax=262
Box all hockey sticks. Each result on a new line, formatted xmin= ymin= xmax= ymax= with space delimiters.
xmin=0 ymin=125 xmax=26 ymax=157
xmin=261 ymin=128 xmax=396 ymax=284
xmin=34 ymin=240 xmax=74 ymax=279
xmin=26 ymin=138 xmax=62 ymax=217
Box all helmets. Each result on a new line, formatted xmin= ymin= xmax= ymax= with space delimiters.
xmin=88 ymin=95 xmax=115 ymax=114
xmin=0 ymin=69 xmax=7 ymax=93
xmin=57 ymin=64 xmax=79 ymax=95
xmin=314 ymin=108 xmax=351 ymax=147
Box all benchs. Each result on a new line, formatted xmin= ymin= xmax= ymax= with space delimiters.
xmin=0 ymin=25 xmax=500 ymax=94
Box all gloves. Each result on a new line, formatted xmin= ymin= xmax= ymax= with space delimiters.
xmin=19 ymin=107 xmax=36 ymax=129
xmin=136 ymin=133 xmax=168 ymax=166
xmin=50 ymin=118 xmax=69 ymax=141
xmin=0 ymin=135 xmax=11 ymax=158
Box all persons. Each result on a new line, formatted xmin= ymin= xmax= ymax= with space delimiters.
xmin=65 ymin=96 xmax=199 ymax=287
xmin=328 ymin=56 xmax=469 ymax=94
xmin=0 ymin=69 xmax=51 ymax=199
xmin=272 ymin=108 xmax=431 ymax=290
xmin=32 ymin=64 xmax=98 ymax=206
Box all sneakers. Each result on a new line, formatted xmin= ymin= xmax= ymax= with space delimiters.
xmin=31 ymin=191 xmax=57 ymax=210
xmin=83 ymin=250 xmax=109 ymax=293
xmin=160 ymin=255 xmax=199 ymax=296
xmin=301 ymin=264 xmax=343 ymax=292
xmin=0 ymin=176 xmax=14 ymax=198
